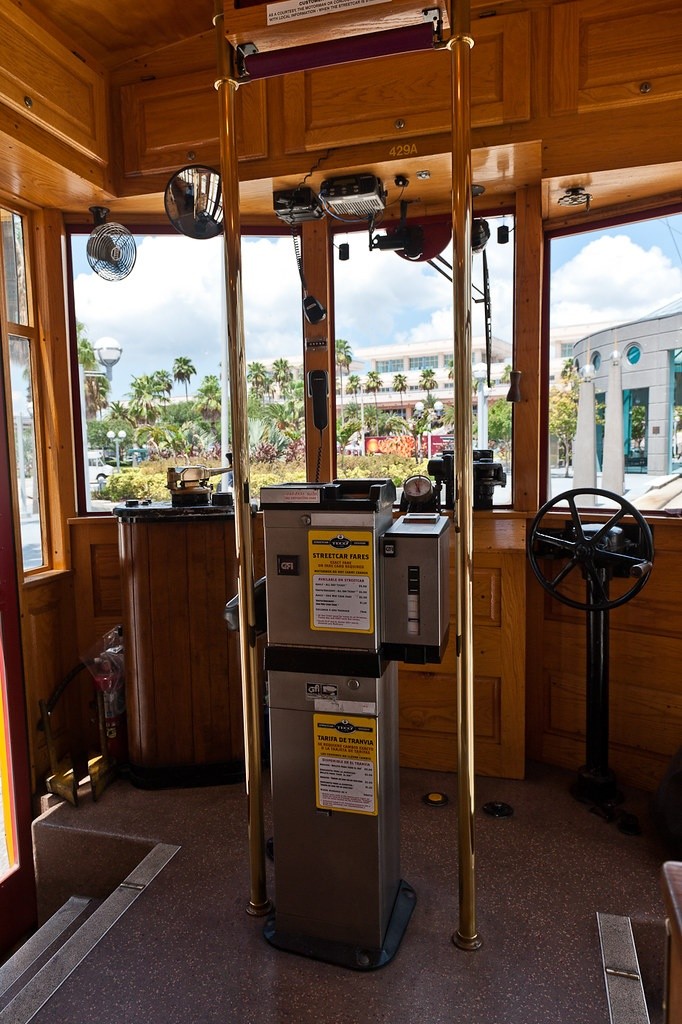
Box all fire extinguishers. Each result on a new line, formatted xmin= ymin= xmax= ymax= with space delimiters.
xmin=36 ymin=625 xmax=127 ymax=759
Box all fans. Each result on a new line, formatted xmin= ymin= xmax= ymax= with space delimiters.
xmin=86 ymin=206 xmax=138 ymax=282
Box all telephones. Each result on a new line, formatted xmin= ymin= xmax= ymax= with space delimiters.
xmin=307 ymin=369 xmax=330 ymax=431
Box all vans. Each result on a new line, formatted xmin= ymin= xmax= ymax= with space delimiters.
xmin=87 ymin=449 xmax=115 ymax=485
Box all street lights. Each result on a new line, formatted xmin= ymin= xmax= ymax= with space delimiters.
xmin=415 ymin=400 xmax=443 ymax=459
xmin=108 ymin=430 xmax=127 ymax=473
